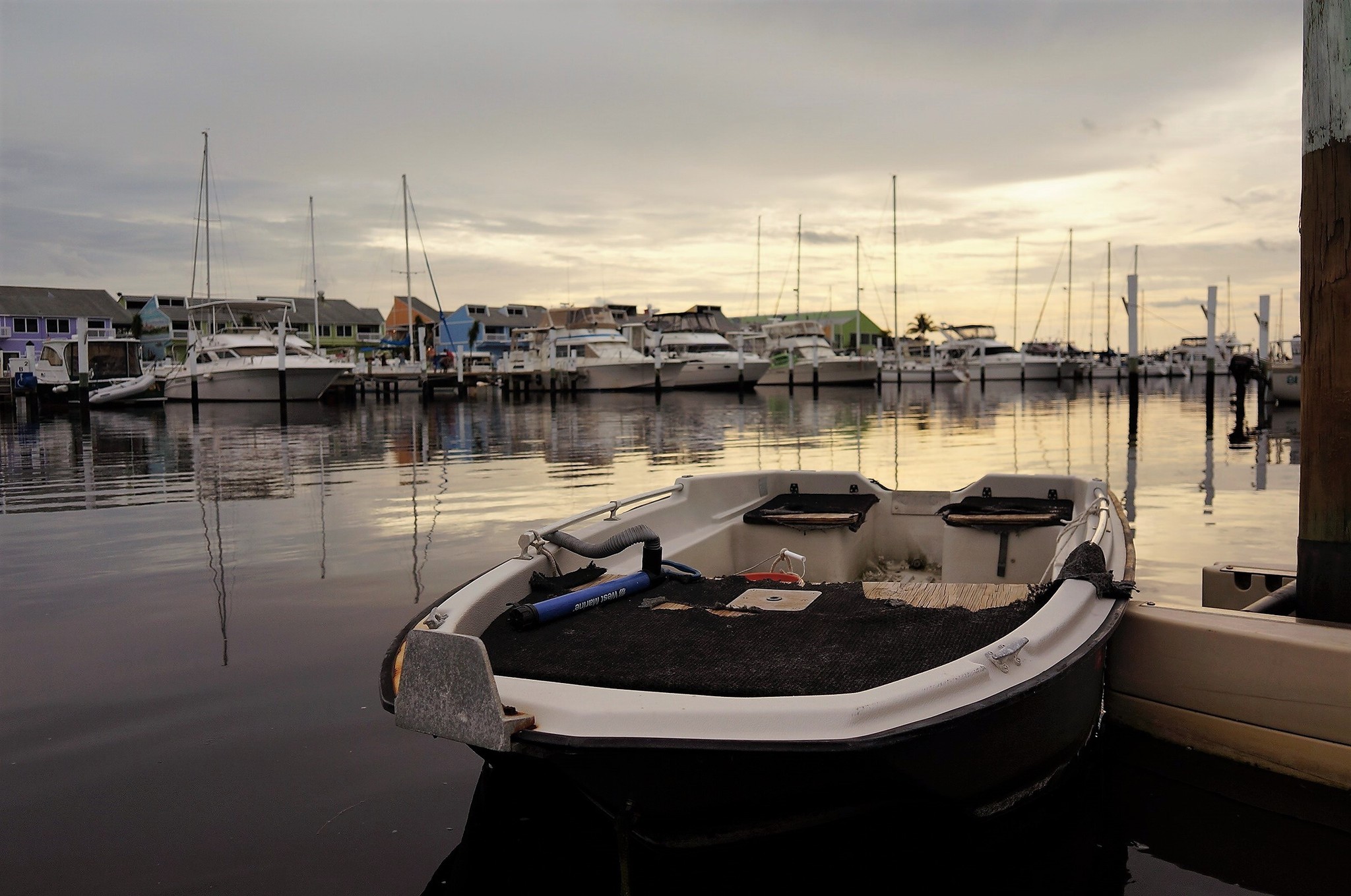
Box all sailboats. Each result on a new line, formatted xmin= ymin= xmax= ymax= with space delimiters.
xmin=871 ymin=174 xmax=1304 ymax=410
xmin=144 ymin=130 xmax=498 ymax=396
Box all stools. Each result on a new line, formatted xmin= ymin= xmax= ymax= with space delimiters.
xmin=739 ymin=492 xmax=879 ymax=578
xmin=939 ymin=495 xmax=1073 ymax=581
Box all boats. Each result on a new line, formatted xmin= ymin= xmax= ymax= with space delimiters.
xmin=737 ymin=320 xmax=879 ymax=388
xmin=376 ymin=469 xmax=1141 ymax=861
xmin=494 ymin=302 xmax=657 ymax=394
xmin=641 ymin=310 xmax=773 ymax=394
xmin=5 ymin=327 xmax=183 ymax=416
xmin=163 ymin=297 xmax=360 ymax=406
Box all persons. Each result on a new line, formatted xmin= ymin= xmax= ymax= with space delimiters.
xmin=430 ymin=349 xmax=454 ymax=373
